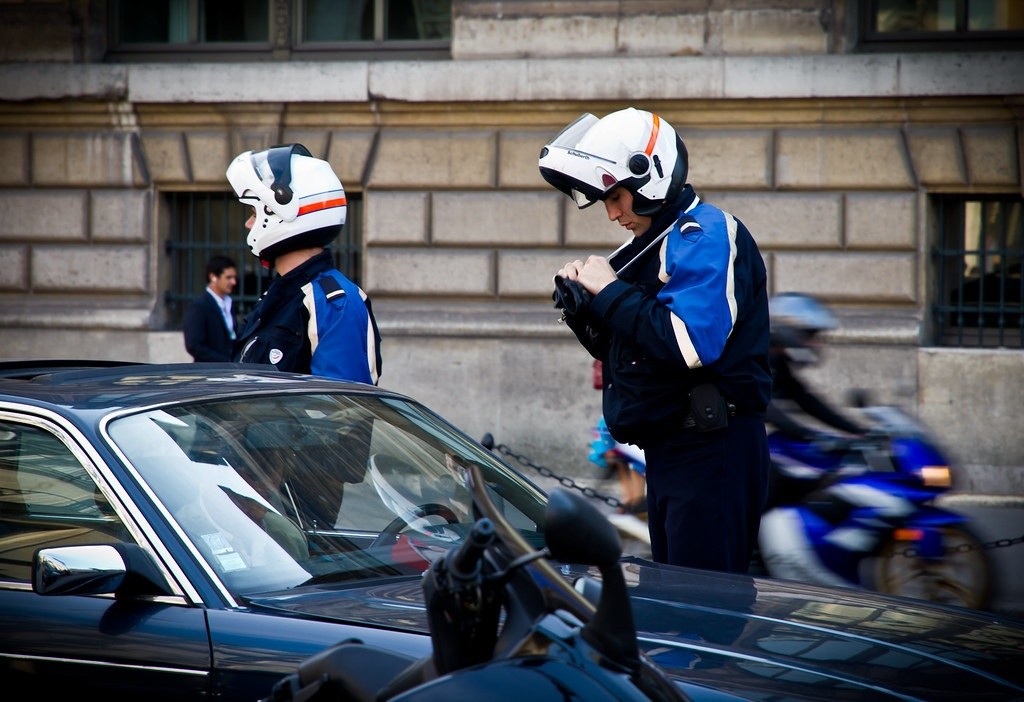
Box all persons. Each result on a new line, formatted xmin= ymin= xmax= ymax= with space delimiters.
xmin=222 ymin=141 xmax=381 ymax=532
xmin=183 ymin=255 xmax=243 ymax=464
xmin=174 ymin=433 xmax=310 ymax=574
xmin=539 ymin=105 xmax=774 ymax=577
xmin=761 ymin=288 xmax=889 ymax=446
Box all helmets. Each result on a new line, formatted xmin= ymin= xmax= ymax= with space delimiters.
xmin=767 ymin=292 xmax=840 ymax=370
xmin=539 ymin=107 xmax=688 ymax=217
xmin=226 ymin=143 xmax=348 ymax=261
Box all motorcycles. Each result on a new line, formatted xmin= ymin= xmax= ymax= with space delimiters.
xmin=582 ymin=382 xmax=1001 ymax=615
xmin=258 ymin=461 xmax=694 ymax=701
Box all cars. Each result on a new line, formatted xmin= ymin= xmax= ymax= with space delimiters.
xmin=0 ymin=359 xmax=1024 ymax=702
